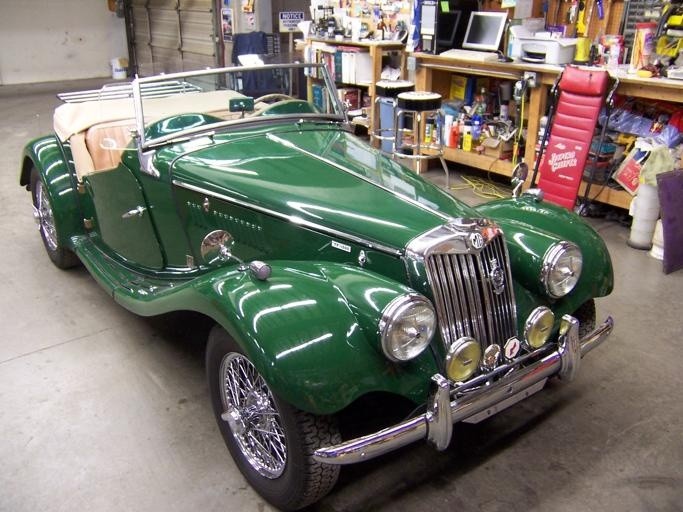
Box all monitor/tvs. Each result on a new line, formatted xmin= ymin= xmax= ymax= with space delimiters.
xmin=580 ymin=129 xmax=621 ymax=185
xmin=533 ymin=115 xmax=551 ymax=171
xmin=302 ymin=44 xmax=373 ymax=121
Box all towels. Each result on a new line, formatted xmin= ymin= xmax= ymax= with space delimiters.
xmin=380 ymin=102 xmax=405 ymax=154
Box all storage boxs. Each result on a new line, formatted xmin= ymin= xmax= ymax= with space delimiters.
xmin=53 ymin=88 xmax=274 ymax=193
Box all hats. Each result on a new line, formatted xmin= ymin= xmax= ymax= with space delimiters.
xmin=19 ymin=56 xmax=614 ymax=512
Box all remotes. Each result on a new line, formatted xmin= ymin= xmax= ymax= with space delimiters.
xmin=505 ymin=56 xmax=514 ymax=62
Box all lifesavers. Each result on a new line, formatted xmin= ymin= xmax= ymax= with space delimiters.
xmin=419 ymin=0 xmax=479 ymax=54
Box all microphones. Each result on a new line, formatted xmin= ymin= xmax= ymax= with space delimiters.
xmin=393 ymin=91 xmax=450 ymax=189
xmin=367 ymin=79 xmax=416 ymax=166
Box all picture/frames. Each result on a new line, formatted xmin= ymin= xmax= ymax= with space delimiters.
xmin=526 ymin=62 xmax=683 ymax=210
xmin=412 ymin=51 xmax=535 ymax=170
xmin=301 ymin=37 xmax=407 ymax=148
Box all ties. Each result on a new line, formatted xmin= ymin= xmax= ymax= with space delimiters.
xmin=462 ymin=11 xmax=508 ymax=51
xmin=436 ymin=10 xmax=462 ymax=46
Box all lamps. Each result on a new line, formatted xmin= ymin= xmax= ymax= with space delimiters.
xmin=229 ymin=32 xmax=288 ymax=98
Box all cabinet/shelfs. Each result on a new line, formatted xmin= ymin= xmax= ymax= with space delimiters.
xmin=424 ymin=115 xmax=481 ymax=152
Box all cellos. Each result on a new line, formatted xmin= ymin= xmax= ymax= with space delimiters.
xmin=511 ymin=39 xmax=575 ymax=64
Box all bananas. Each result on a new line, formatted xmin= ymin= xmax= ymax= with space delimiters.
xmin=440 ymin=48 xmax=499 ymax=63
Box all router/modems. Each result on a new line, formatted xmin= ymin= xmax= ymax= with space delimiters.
xmin=334 ymin=35 xmax=344 ymax=40
